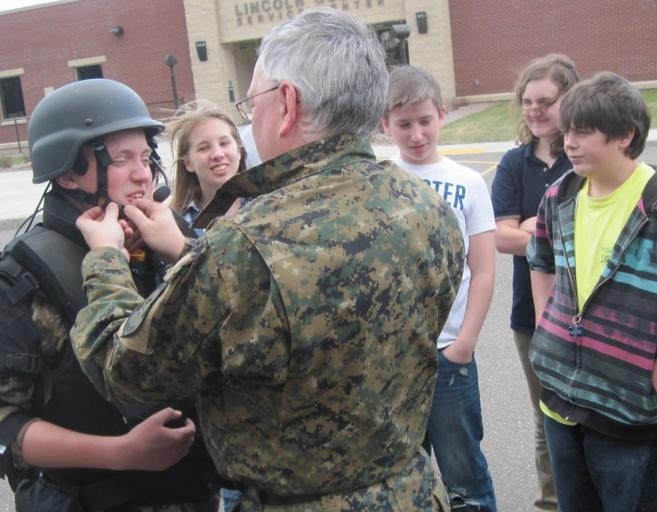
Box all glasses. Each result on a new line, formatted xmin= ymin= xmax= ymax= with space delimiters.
xmin=235 ymin=86 xmax=282 ymax=123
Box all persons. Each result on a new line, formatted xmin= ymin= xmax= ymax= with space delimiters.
xmin=159 ymin=99 xmax=250 ymax=233
xmin=489 ymin=52 xmax=584 ymax=511
xmin=71 ymin=3 xmax=463 ymax=512
xmin=0 ymin=77 xmax=224 ymax=512
xmin=375 ymin=64 xmax=500 ymax=511
xmin=523 ymin=70 xmax=657 ymax=511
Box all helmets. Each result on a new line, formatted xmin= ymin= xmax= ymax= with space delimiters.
xmin=26 ymin=79 xmax=167 ymax=184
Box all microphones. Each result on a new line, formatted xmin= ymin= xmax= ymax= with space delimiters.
xmin=151 ymin=157 xmax=171 ymax=202
